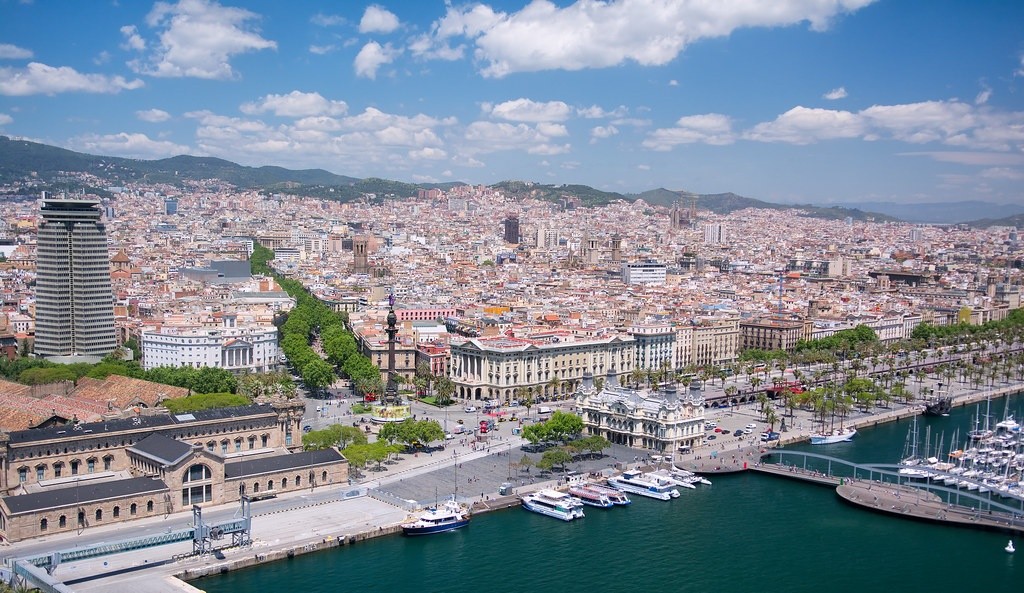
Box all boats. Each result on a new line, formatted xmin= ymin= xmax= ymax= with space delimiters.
xmin=807 ymin=427 xmax=857 ymax=446
xmin=569 ymin=465 xmax=712 ymax=508
xmin=401 ymin=493 xmax=472 ymax=536
xmin=522 ymin=487 xmax=586 ymax=522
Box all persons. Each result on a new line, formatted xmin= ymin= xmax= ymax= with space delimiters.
xmin=389 ymin=293 xmax=396 ymax=309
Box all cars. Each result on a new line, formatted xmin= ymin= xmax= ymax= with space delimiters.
xmin=453 ymin=406 xmax=552 ymax=437
xmin=705 ymin=420 xmax=781 ymax=443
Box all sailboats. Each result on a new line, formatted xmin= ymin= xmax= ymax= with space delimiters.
xmin=894 ymin=382 xmax=1024 ymax=504
